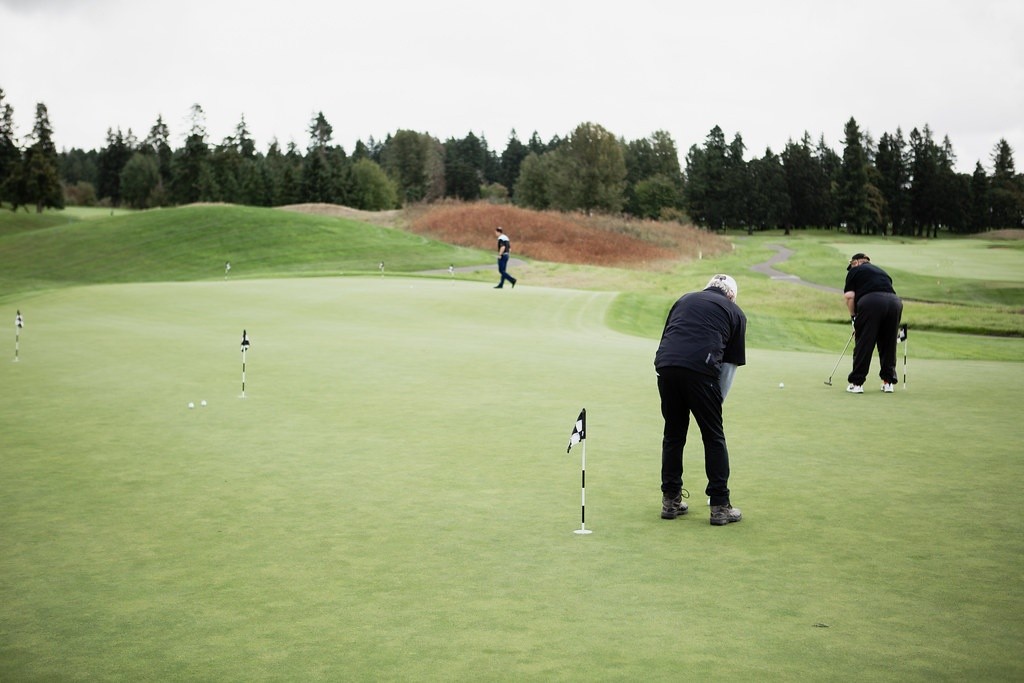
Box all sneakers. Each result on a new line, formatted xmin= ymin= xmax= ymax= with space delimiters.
xmin=710 ymin=503 xmax=741 ymax=525
xmin=881 ymin=381 xmax=893 ymax=392
xmin=847 ymin=383 xmax=864 ymax=393
xmin=662 ymin=489 xmax=690 ymax=518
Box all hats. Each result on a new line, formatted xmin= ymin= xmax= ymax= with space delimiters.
xmin=847 ymin=253 xmax=869 ymax=270
xmin=710 ymin=274 xmax=738 ymax=296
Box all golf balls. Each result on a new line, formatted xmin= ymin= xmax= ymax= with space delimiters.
xmin=779 ymin=382 xmax=785 ymax=388
xmin=707 ymin=498 xmax=711 ymax=505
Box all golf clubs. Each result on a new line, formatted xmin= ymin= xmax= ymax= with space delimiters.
xmin=823 ymin=333 xmax=854 ymax=386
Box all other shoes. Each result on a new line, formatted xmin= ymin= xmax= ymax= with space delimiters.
xmin=512 ymin=279 xmax=516 ymax=287
xmin=494 ymin=286 xmax=502 ymax=288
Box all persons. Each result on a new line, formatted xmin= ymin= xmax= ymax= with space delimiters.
xmin=654 ymin=274 xmax=748 ymax=526
xmin=493 ymin=227 xmax=516 ymax=289
xmin=843 ymin=253 xmax=903 ymax=393
